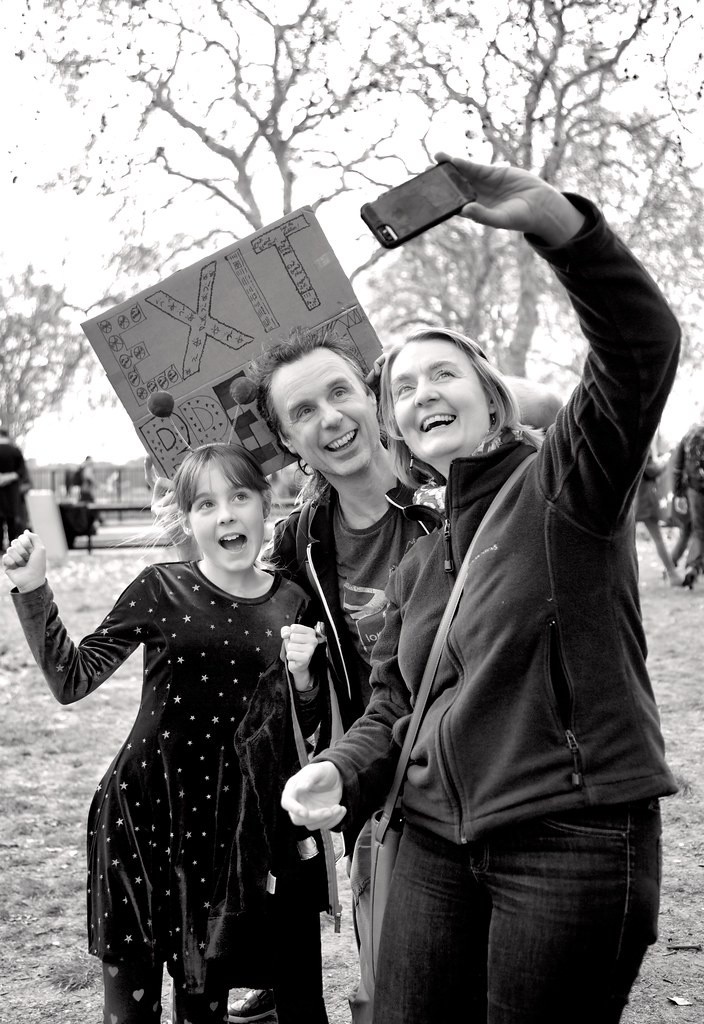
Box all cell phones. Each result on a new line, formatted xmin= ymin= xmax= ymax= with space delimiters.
xmin=359 ymin=158 xmax=476 ymax=248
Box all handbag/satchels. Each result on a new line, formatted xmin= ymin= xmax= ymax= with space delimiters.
xmin=348 ymin=809 xmax=400 ymax=1024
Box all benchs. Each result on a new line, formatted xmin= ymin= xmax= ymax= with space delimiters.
xmin=87 ymin=502 xmax=154 ymax=551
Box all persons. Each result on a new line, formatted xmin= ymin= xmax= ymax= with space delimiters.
xmin=0 ymin=430 xmax=31 ymax=555
xmin=76 ymin=454 xmax=105 ymax=526
xmin=149 ymin=329 xmax=564 ymax=1023
xmin=279 ymin=147 xmax=683 ymax=1024
xmin=2 ymin=442 xmax=330 ymax=1024
xmin=634 ymin=423 xmax=704 ymax=589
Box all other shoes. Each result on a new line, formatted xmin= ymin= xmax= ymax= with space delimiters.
xmin=685 ymin=566 xmax=697 ymax=589
xmin=670 ymin=574 xmax=685 ymax=585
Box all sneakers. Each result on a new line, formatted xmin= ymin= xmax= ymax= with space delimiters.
xmin=227 ymin=989 xmax=276 ymax=1023
